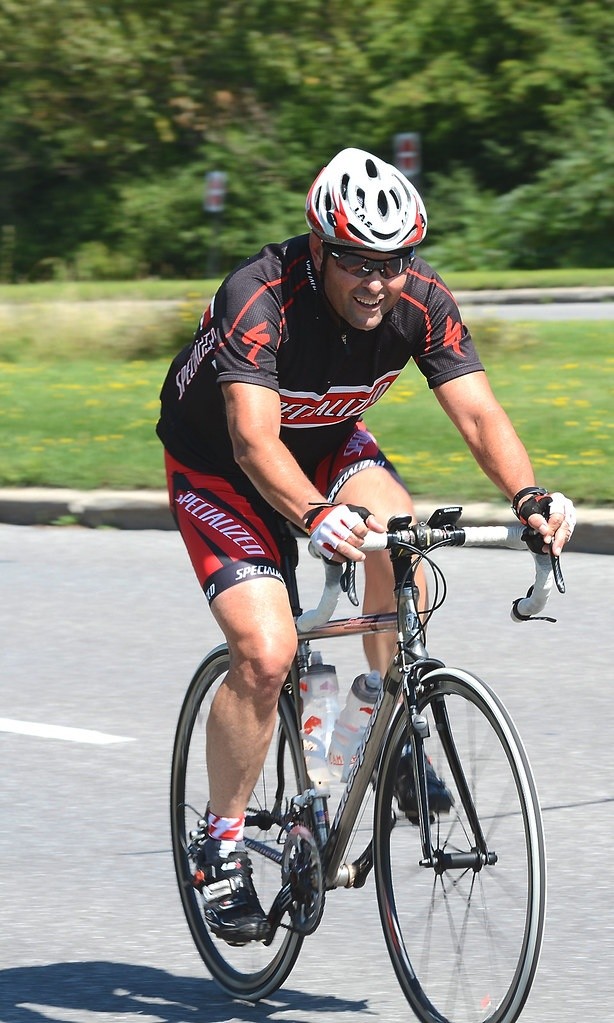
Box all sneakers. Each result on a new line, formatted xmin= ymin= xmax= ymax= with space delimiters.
xmin=369 ymin=739 xmax=453 ymax=818
xmin=195 ymin=835 xmax=271 ymax=940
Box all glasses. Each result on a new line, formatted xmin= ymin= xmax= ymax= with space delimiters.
xmin=321 ymin=240 xmax=415 ymax=279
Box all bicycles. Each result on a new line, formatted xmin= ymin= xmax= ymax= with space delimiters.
xmin=167 ymin=499 xmax=571 ymax=1023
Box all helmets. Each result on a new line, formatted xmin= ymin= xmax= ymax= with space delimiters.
xmin=305 ymin=148 xmax=428 ymax=251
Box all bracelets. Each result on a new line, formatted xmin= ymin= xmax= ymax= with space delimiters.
xmin=510 ymin=486 xmax=548 ymax=515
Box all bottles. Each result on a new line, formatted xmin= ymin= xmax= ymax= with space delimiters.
xmin=296 ymin=650 xmax=341 ymax=783
xmin=326 ymin=668 xmax=383 ymax=782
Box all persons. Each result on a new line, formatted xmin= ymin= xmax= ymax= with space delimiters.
xmin=158 ymin=147 xmax=579 ymax=943
